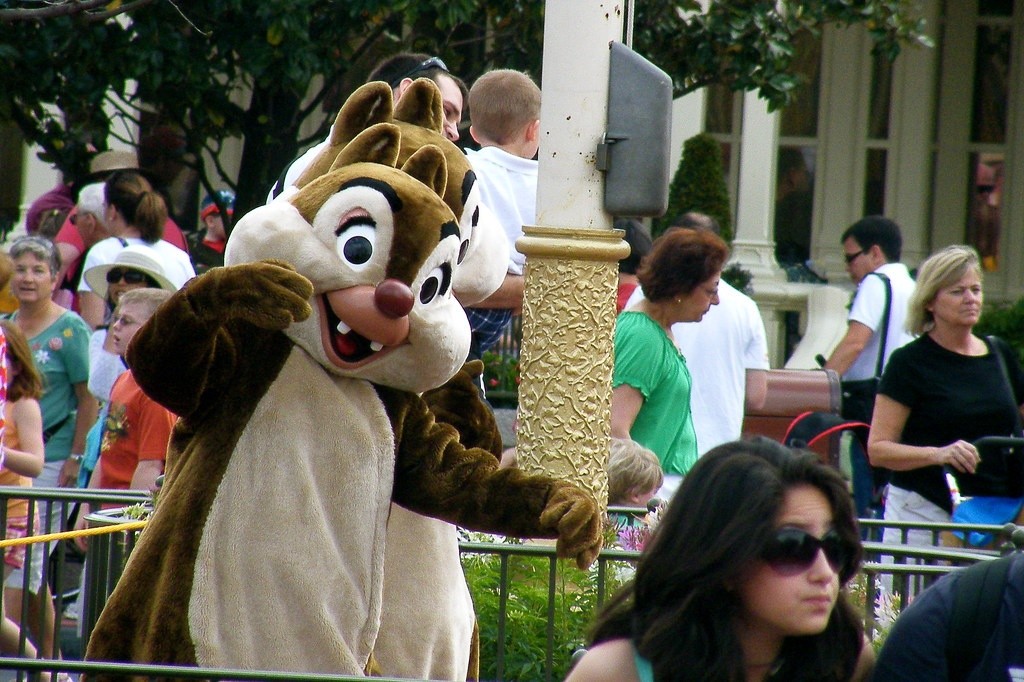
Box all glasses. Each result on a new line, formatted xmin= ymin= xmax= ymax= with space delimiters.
xmin=763 ymin=524 xmax=848 ymax=576
xmin=388 ymin=56 xmax=449 ymax=88
xmin=114 ymin=317 xmax=140 ymax=327
xmin=843 ymin=247 xmax=869 ymax=264
xmin=698 ymin=282 xmax=718 ymax=298
xmin=68 ymin=212 xmax=92 ymax=225
xmin=106 ymin=268 xmax=145 ymax=286
xmin=12 ymin=236 xmax=54 ymax=276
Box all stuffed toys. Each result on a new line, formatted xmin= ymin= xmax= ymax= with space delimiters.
xmin=79 ymin=78 xmax=604 ymax=682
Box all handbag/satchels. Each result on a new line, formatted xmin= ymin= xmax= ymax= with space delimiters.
xmin=838 ymin=377 xmax=878 ymax=425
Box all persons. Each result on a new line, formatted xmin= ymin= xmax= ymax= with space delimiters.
xmin=866 ymin=244 xmax=1024 ymax=636
xmin=825 ymin=216 xmax=919 ymax=542
xmin=608 ymin=437 xmax=663 ymax=507
xmin=872 ymin=550 xmax=1024 ymax=682
xmin=69 ymin=182 xmax=112 ymax=246
xmin=196 ymin=187 xmax=236 ymax=268
xmin=615 ymin=226 xmax=729 ymax=502
xmin=83 ymin=245 xmax=178 ymax=403
xmin=0 ymin=318 xmax=72 ymax=682
xmin=625 ymin=212 xmax=769 ymax=459
xmin=464 ymin=68 xmax=542 ymax=356
xmin=0 ymin=235 xmax=99 ymax=555
xmin=73 ymin=288 xmax=178 ymax=638
xmin=265 ymin=54 xmax=525 ymax=316
xmin=77 ymin=172 xmax=196 ymax=332
xmin=25 ymin=135 xmax=96 ymax=238
xmin=56 ymin=151 xmax=189 ymax=288
xmin=617 ymin=219 xmax=651 ymax=314
xmin=566 ymin=436 xmax=877 ymax=682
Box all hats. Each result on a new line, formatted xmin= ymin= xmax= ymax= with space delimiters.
xmin=82 ymin=243 xmax=181 ymax=301
xmin=201 ymin=191 xmax=237 ymax=221
xmin=78 ymin=150 xmax=140 ymax=187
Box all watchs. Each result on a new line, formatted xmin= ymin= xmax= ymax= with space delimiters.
xmin=71 ymin=453 xmax=84 ymax=465
